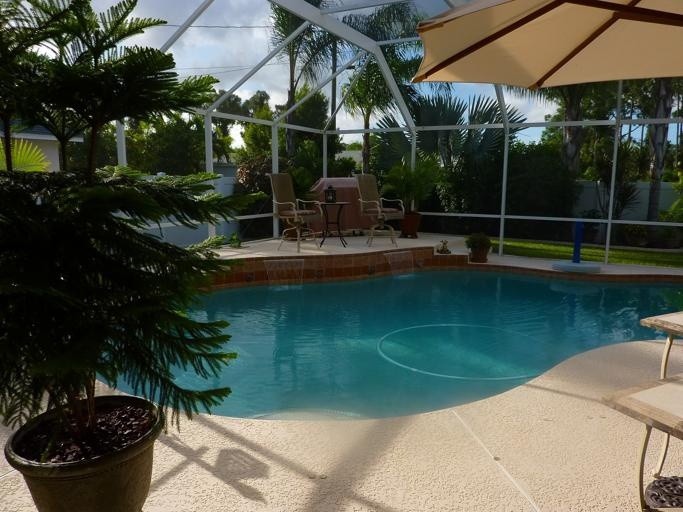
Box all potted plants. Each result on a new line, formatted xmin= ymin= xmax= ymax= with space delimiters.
xmin=463 ymin=232 xmax=493 ymax=263
xmin=1 ymin=0 xmax=243 ymax=511
xmin=377 ymin=150 xmax=443 ymax=239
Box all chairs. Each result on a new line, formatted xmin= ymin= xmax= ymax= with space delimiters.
xmin=265 ymin=172 xmax=407 ymax=254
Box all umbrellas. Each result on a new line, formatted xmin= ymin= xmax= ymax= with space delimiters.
xmin=411 ymin=0 xmax=683 ymax=90
